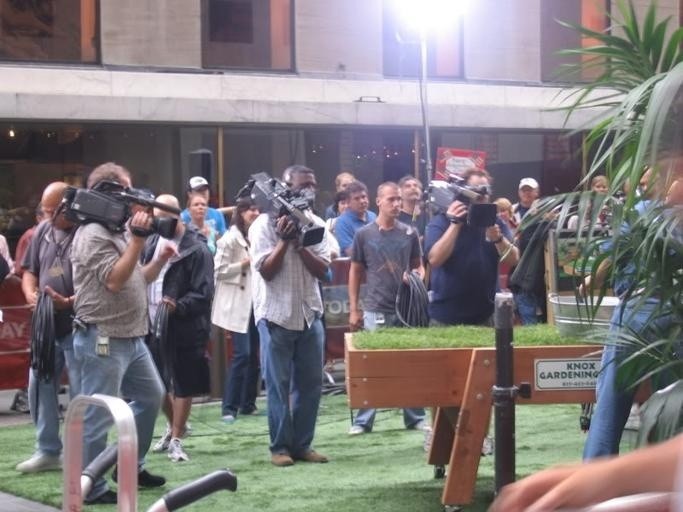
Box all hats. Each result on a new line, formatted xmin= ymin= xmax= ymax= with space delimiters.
xmin=520 ymin=176 xmax=539 ymax=192
xmin=185 ymin=176 xmax=209 ymax=192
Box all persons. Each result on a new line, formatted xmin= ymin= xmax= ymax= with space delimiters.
xmin=479 ymin=427 xmax=683 ymax=512
xmin=0 ymin=181 xmax=81 ymax=472
xmin=124 ymin=153 xmax=683 ymax=466
xmin=66 ymin=161 xmax=186 ymax=507
